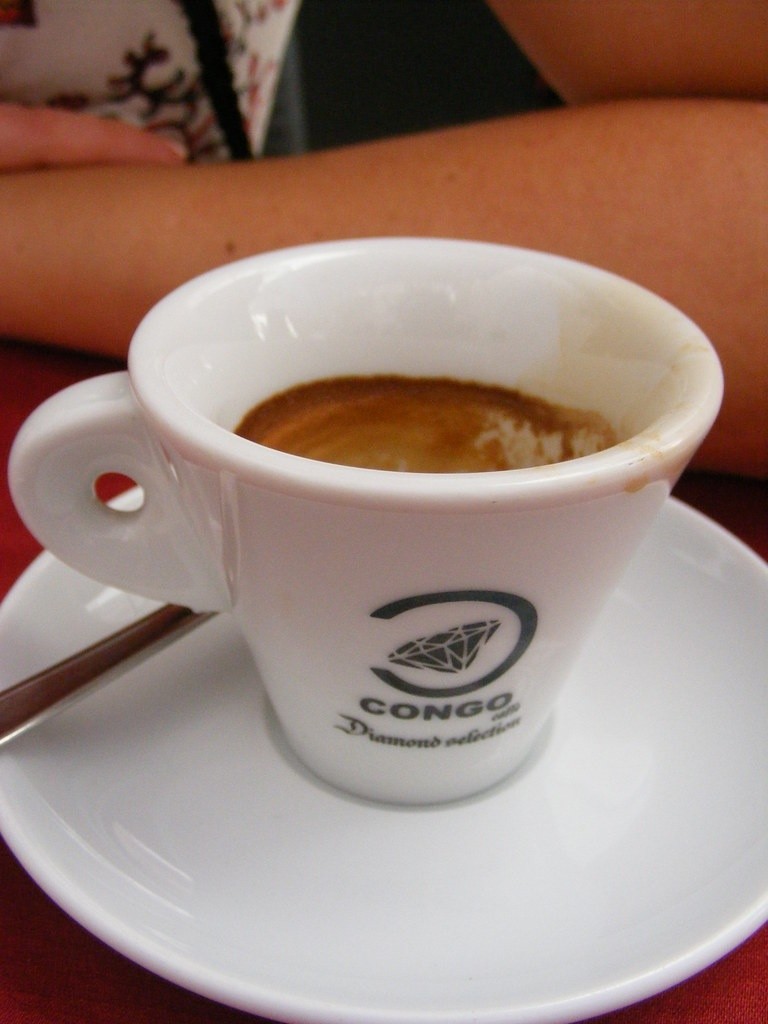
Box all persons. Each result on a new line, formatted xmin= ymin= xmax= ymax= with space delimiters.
xmin=0 ymin=0 xmax=768 ymax=484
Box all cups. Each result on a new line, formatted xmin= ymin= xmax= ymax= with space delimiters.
xmin=5 ymin=237 xmax=726 ymax=809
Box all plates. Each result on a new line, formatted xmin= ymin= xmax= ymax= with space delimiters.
xmin=0 ymin=464 xmax=766 ymax=1022
